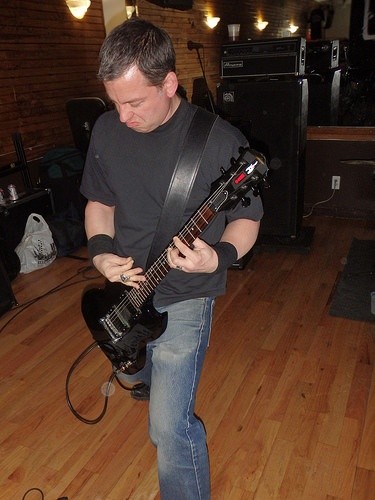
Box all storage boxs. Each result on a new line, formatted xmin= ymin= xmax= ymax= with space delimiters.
xmin=0 ymin=188 xmax=55 ymax=244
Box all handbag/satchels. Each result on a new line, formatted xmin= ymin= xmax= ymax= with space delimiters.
xmin=15 ymin=213 xmax=57 ymax=273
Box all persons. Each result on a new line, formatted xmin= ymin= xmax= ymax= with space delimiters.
xmin=78 ymin=19 xmax=265 ymax=500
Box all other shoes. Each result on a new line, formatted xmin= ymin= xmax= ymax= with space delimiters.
xmin=131 ymin=383 xmax=150 ymax=400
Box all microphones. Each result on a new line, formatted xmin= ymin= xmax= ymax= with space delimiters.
xmin=187 ymin=41 xmax=203 ymax=51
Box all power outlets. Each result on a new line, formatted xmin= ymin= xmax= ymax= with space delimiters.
xmin=332 ymin=175 xmax=341 ymax=190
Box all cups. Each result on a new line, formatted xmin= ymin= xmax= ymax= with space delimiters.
xmin=227 ymin=24 xmax=240 ymax=44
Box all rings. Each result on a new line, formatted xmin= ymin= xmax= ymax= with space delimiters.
xmin=120 ymin=274 xmax=130 ymax=282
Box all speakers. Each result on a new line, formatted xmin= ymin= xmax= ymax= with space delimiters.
xmin=217 ymin=78 xmax=309 ymax=241
xmin=305 ymin=70 xmax=343 ymax=126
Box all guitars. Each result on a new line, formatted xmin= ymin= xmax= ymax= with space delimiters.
xmin=80 ymin=146 xmax=272 ymax=376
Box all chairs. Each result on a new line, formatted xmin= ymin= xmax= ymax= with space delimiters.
xmin=66 ymin=95 xmax=108 ymax=152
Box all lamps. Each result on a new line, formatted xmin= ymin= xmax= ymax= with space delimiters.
xmin=203 ymin=17 xmax=220 ymax=30
xmin=254 ymin=22 xmax=269 ymax=31
xmin=66 ymin=0 xmax=91 ymax=19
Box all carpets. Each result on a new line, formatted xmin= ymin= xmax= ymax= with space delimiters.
xmin=327 ymin=236 xmax=375 ymax=322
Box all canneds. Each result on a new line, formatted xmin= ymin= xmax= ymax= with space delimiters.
xmin=7 ymin=184 xmax=19 ymax=200
xmin=0 ymin=189 xmax=6 ymax=205
